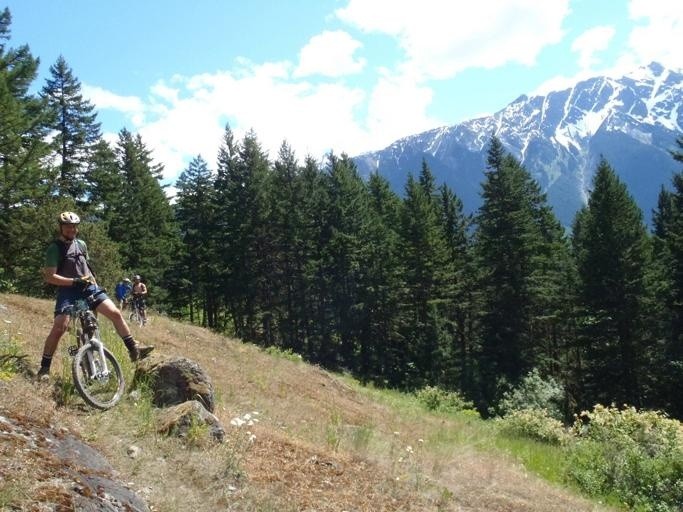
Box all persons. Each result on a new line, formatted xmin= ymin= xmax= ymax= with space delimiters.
xmin=131 ymin=275 xmax=147 ymax=322
xmin=115 ymin=278 xmax=132 ymax=312
xmin=37 ymin=211 xmax=155 ymax=382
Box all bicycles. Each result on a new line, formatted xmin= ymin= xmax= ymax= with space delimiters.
xmin=51 ymin=289 xmax=127 ymax=410
xmin=115 ymin=293 xmax=152 ymax=328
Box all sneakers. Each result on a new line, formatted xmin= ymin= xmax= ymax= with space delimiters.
xmin=37 ymin=366 xmax=50 ymax=385
xmin=130 ymin=345 xmax=155 ymax=362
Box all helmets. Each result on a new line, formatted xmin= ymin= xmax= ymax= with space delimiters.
xmin=134 ymin=275 xmax=141 ymax=280
xmin=123 ymin=278 xmax=129 ymax=282
xmin=58 ymin=212 xmax=81 ymax=225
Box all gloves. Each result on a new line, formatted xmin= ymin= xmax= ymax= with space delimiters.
xmin=72 ymin=275 xmax=96 ymax=288
